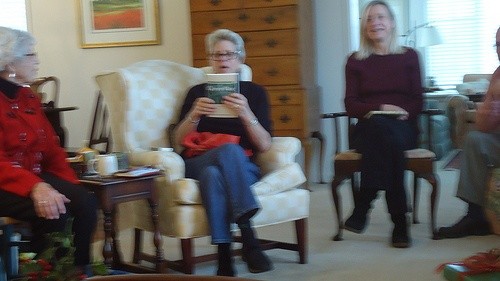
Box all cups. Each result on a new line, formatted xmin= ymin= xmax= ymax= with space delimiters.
xmin=87 ymin=155 xmax=117 ymax=178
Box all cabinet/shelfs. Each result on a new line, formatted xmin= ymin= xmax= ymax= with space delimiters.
xmin=190 ymin=0 xmax=318 ymax=192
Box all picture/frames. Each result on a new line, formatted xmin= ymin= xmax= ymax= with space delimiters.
xmin=75 ymin=0 xmax=160 ymax=49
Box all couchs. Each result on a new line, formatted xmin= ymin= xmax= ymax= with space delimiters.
xmin=449 ymin=74 xmax=494 ymax=149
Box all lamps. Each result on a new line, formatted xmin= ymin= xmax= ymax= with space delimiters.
xmin=401 ymin=20 xmax=441 ymax=48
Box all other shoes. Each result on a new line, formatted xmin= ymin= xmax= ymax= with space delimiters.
xmin=392 ymin=218 xmax=412 ymax=248
xmin=343 ymin=203 xmax=368 ymax=234
xmin=240 ymin=238 xmax=275 ymax=273
xmin=217 ymin=252 xmax=236 ymax=278
xmin=438 ymin=214 xmax=491 ymax=238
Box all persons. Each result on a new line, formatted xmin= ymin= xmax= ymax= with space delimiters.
xmin=434 ymin=27 xmax=500 ymax=238
xmin=343 ymin=0 xmax=423 ymax=248
xmin=170 ymin=28 xmax=274 ymax=276
xmin=0 ymin=26 xmax=97 ymax=269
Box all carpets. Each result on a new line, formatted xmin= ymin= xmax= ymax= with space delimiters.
xmin=441 ymin=151 xmax=462 ymax=171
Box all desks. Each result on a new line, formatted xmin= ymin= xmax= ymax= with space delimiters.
xmin=65 ymin=152 xmax=166 ymax=276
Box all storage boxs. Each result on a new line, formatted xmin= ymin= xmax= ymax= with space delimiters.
xmin=443 ymin=248 xmax=500 ymax=281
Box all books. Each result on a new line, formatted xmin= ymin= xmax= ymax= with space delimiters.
xmin=364 ymin=110 xmax=407 ymax=119
xmin=205 ymin=73 xmax=240 ymax=118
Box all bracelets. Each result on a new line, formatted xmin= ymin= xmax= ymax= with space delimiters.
xmin=241 ymin=119 xmax=258 ymax=127
xmin=191 ymin=117 xmax=200 ymax=126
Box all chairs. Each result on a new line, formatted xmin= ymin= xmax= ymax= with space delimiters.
xmin=95 ymin=59 xmax=310 ymax=275
xmin=322 ymin=108 xmax=444 ymax=240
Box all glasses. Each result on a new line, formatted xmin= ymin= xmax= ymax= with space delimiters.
xmin=22 ymin=52 xmax=39 ymax=58
xmin=206 ymin=51 xmax=240 ymax=61
